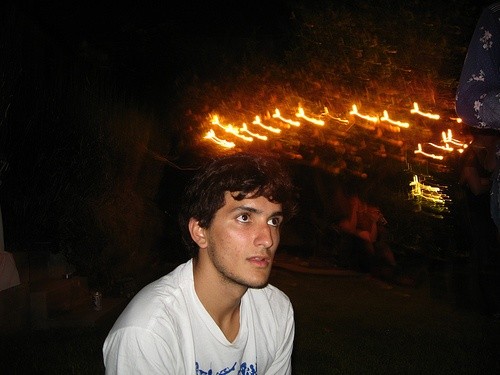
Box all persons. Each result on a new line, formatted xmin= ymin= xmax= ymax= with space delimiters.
xmin=103 ymin=153 xmax=295 ymax=375
xmin=1 ymin=250 xmax=40 ymax=312
xmin=282 ymin=147 xmax=499 ymax=282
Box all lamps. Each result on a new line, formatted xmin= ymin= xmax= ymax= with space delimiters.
xmin=185 ymin=0 xmax=480 ymax=222
